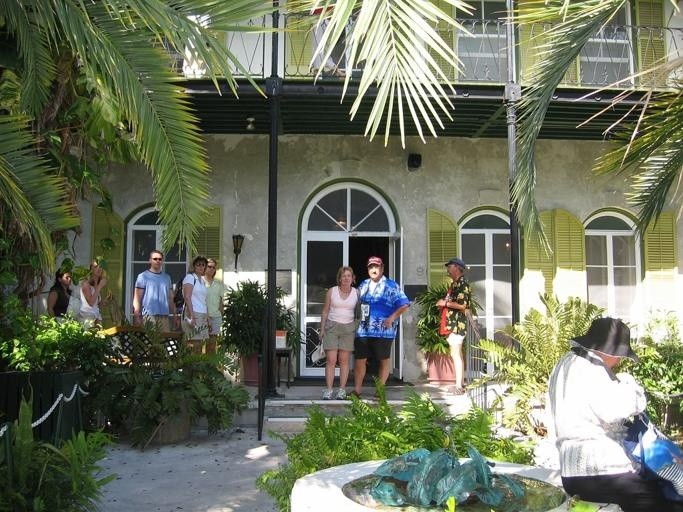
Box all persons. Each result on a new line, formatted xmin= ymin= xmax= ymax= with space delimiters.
xmin=548 ymin=317 xmax=682 ymax=511
xmin=311 ymin=50 xmax=351 ymax=80
xmin=180 ymin=256 xmax=208 ymax=376
xmin=318 ymin=266 xmax=358 ymax=400
xmin=436 ymin=259 xmax=470 ymax=395
xmin=345 ymin=257 xmax=410 ymax=400
xmin=47 ymin=268 xmax=72 ymax=324
xmin=132 ymin=250 xmax=180 ymax=367
xmin=202 ymin=257 xmax=225 ymax=355
xmin=77 ymin=260 xmax=110 ymax=332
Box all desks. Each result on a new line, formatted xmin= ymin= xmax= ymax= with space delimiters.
xmin=275 ymin=348 xmax=292 ymax=389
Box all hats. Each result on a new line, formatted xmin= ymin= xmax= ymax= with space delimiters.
xmin=311 ymin=339 xmax=326 ymax=362
xmin=445 ymin=259 xmax=466 ymax=268
xmin=570 ymin=318 xmax=639 ymax=362
xmin=368 ymin=256 xmax=383 ymax=266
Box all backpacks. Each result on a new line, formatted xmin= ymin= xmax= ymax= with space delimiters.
xmin=174 ymin=273 xmax=195 ymax=307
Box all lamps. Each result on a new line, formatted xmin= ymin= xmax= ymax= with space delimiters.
xmin=232 ymin=232 xmax=245 ymax=272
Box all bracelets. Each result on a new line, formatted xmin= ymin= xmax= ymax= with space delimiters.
xmin=444 ymin=300 xmax=448 ymax=308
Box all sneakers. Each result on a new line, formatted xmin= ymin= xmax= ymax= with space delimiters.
xmin=447 ymin=387 xmax=464 ymax=396
xmin=323 ymin=390 xmax=380 ymax=400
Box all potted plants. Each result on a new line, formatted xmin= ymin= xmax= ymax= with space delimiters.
xmin=0 ymin=293 xmax=123 ymax=488
xmin=216 ymin=278 xmax=307 ymax=387
xmin=411 ymin=279 xmax=485 ymax=386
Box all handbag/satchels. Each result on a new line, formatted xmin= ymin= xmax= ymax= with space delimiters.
xmin=624 ymin=412 xmax=683 ymax=496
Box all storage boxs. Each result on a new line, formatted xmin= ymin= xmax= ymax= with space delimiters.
xmin=275 ymin=329 xmax=288 ymax=348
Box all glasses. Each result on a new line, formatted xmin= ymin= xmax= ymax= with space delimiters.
xmin=153 ymin=258 xmax=161 ymax=260
